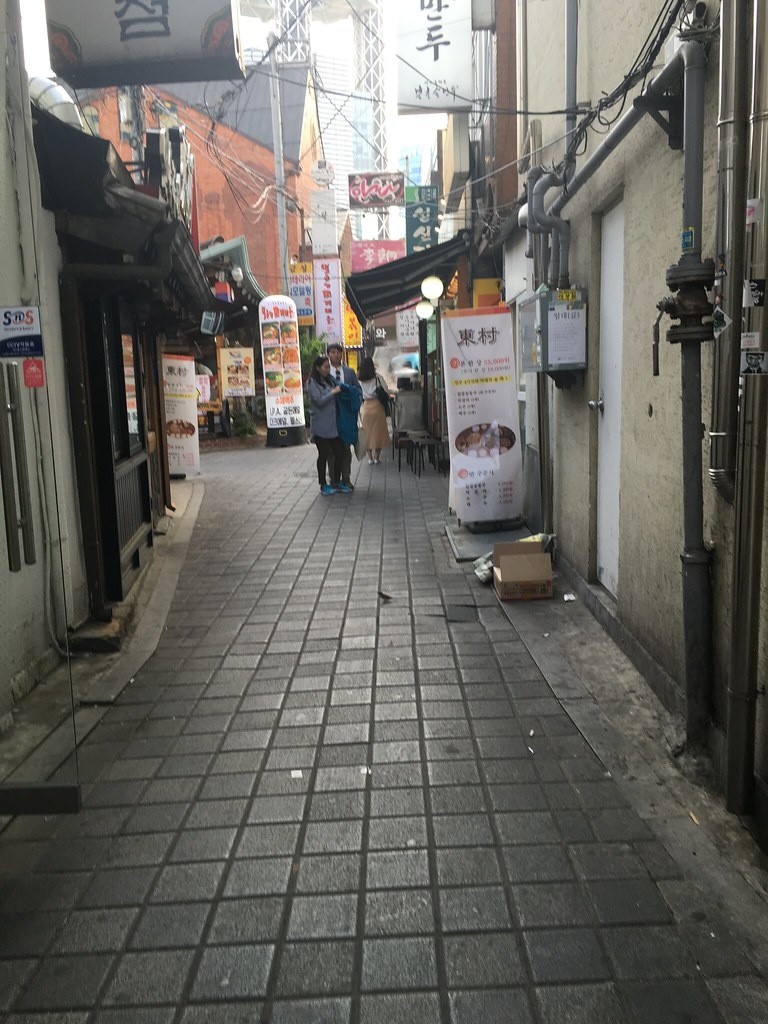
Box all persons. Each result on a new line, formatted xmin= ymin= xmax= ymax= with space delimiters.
xmin=306 ymin=344 xmax=392 ymax=496
xmin=387 ymin=361 xmax=419 ymax=391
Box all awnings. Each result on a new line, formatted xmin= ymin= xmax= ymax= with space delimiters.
xmin=346 ymin=229 xmax=472 ymax=328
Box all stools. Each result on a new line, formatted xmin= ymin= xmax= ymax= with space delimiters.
xmin=397 ymin=430 xmax=444 ymax=479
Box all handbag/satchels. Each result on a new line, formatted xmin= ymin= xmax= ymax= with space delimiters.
xmin=376 ymin=378 xmax=389 ymax=404
xmin=353 ymin=428 xmax=366 ymax=461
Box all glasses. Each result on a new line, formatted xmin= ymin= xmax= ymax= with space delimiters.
xmin=335 ymin=370 xmax=341 ymax=381
xmin=318 ymin=353 xmax=326 ymax=359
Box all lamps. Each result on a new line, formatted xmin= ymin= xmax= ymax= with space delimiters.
xmin=420 ymin=268 xmax=444 ymax=299
xmin=416 ymin=297 xmax=433 ymax=320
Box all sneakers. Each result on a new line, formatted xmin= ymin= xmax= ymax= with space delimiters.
xmin=320 ymin=483 xmax=335 ymax=496
xmin=330 ymin=480 xmax=352 ymax=493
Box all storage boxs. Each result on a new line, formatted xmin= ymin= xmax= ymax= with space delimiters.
xmin=489 ymin=542 xmax=553 ymax=600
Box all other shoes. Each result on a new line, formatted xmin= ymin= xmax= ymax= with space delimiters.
xmin=342 ymin=480 xmax=354 ymax=489
xmin=368 ymin=459 xmax=372 ymax=464
xmin=375 ymin=460 xmax=380 ymax=465
